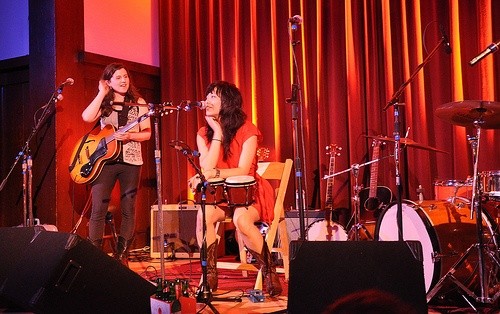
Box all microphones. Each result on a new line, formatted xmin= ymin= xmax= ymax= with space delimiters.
xmin=441 ymin=28 xmax=452 ymax=55
xmin=61 ymin=78 xmax=74 ymax=86
xmin=289 ymin=15 xmax=304 ymax=26
xmin=185 ymin=99 xmax=208 ymax=110
xmin=175 ymin=145 xmax=202 ymax=158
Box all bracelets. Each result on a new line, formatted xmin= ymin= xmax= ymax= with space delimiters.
xmin=213 ymin=167 xmax=220 ymax=178
xmin=211 ymin=138 xmax=223 ymax=143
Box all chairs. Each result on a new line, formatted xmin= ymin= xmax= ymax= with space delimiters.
xmin=198 ymin=159 xmax=293 ymax=293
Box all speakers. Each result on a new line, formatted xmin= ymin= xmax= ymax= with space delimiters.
xmin=288 ymin=240 xmax=428 ymax=314
xmin=0 ymin=227 xmax=157 ymax=314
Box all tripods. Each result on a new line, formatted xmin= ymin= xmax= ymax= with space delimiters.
xmin=180 ymin=148 xmax=243 ymax=314
xmin=426 ymin=116 xmax=500 ymax=314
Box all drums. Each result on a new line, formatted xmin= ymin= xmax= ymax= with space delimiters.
xmin=224 ymin=175 xmax=257 ymax=206
xmin=434 ymin=178 xmax=480 ymax=200
xmin=348 ymin=221 xmax=376 ymax=240
xmin=477 ymin=169 xmax=500 ymax=196
xmin=193 ymin=177 xmax=225 ymax=205
xmin=373 ymin=196 xmax=500 ymax=306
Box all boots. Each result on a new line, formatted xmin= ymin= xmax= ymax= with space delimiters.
xmin=244 ymin=238 xmax=282 ymax=296
xmin=113 ymin=236 xmax=134 ymax=269
xmin=85 ymin=236 xmax=102 ymax=249
xmin=199 ymin=238 xmax=218 ymax=292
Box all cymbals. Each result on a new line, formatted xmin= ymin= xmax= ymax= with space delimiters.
xmin=434 ymin=99 xmax=500 ymax=130
xmin=361 ymin=135 xmax=451 ymax=155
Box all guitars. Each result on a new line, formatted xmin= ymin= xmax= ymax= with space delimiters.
xmin=304 ymin=143 xmax=349 ymax=241
xmin=68 ymin=101 xmax=179 ymax=185
xmin=356 ymin=138 xmax=393 ymax=220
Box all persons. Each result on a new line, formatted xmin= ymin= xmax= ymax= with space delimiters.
xmin=186 ymin=80 xmax=283 ymax=299
xmin=81 ymin=62 xmax=152 ymax=264
